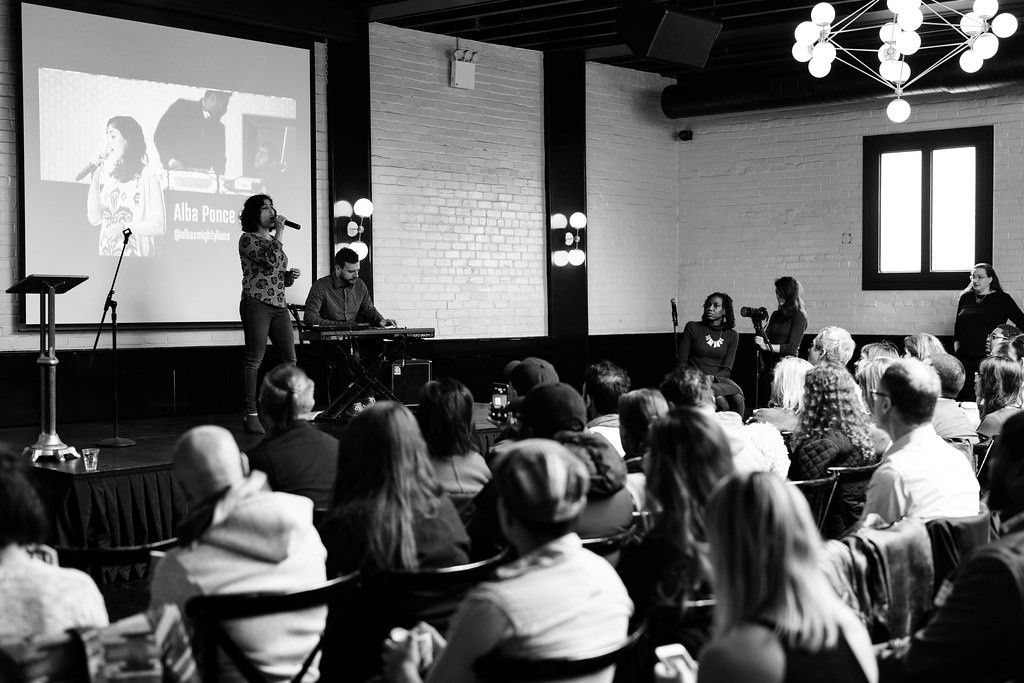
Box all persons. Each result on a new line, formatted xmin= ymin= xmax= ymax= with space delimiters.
xmin=154 ymin=90 xmax=232 ymax=174
xmin=954 ymin=263 xmax=1024 ymax=402
xmin=755 ymin=276 xmax=808 ymax=401
xmin=0 ymin=326 xmax=1024 ymax=683
xmin=86 ymin=116 xmax=166 ymax=256
xmin=677 ymin=292 xmax=745 ymax=417
xmin=304 ymin=249 xmax=397 ymax=411
xmin=238 ymin=193 xmax=300 ymax=433
xmin=254 ymin=141 xmax=277 ymax=168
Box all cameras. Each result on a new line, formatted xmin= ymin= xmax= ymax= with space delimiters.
xmin=741 ymin=305 xmax=768 ymax=320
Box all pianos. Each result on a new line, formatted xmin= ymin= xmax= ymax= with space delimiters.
xmin=299 ymin=321 xmax=436 ymax=424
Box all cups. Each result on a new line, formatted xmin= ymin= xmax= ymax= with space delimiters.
xmin=82 ymin=448 xmax=100 ymax=471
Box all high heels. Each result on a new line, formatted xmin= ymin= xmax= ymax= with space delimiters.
xmin=242 ymin=414 xmax=265 ymax=434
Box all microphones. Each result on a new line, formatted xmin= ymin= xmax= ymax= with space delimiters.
xmin=273 ymin=216 xmax=300 ymax=230
xmin=75 ymin=152 xmax=109 ymax=182
xmin=671 ymin=298 xmax=678 ymax=326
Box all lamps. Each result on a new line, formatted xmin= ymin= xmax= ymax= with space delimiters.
xmin=551 ymin=213 xmax=571 ymax=267
xmin=334 ymin=199 xmax=352 ymax=257
xmin=450 ymin=49 xmax=479 ymax=90
xmin=793 ymin=0 xmax=1018 ymax=124
xmin=348 ymin=198 xmax=373 ymax=262
xmin=568 ymin=212 xmax=589 ymax=267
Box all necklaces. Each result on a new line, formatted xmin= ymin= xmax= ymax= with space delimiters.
xmin=975 ymin=294 xmax=987 ymax=303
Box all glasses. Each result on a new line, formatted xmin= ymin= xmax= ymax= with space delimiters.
xmin=869 ymin=390 xmax=894 ymax=407
xmin=988 ymin=334 xmax=1010 ymax=340
xmin=970 ymin=275 xmax=988 ymax=280
xmin=974 ymin=372 xmax=986 ymax=380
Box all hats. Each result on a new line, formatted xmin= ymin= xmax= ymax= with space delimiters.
xmin=510 ymin=382 xmax=587 ymax=423
xmin=490 ymin=437 xmax=591 ymax=525
xmin=551 ymin=429 xmax=628 ymax=496
xmin=504 ymin=358 xmax=558 ymax=392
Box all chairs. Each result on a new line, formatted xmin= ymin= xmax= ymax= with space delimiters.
xmin=92 ymin=416 xmax=1024 ymax=683
xmin=286 ymin=303 xmax=433 ymax=407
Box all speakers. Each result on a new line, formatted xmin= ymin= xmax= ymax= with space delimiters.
xmin=392 ymin=359 xmax=432 ymax=408
xmin=617 ymin=0 xmax=723 ymax=67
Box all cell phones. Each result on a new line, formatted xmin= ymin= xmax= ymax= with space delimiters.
xmin=491 ymin=383 xmax=510 ymax=422
